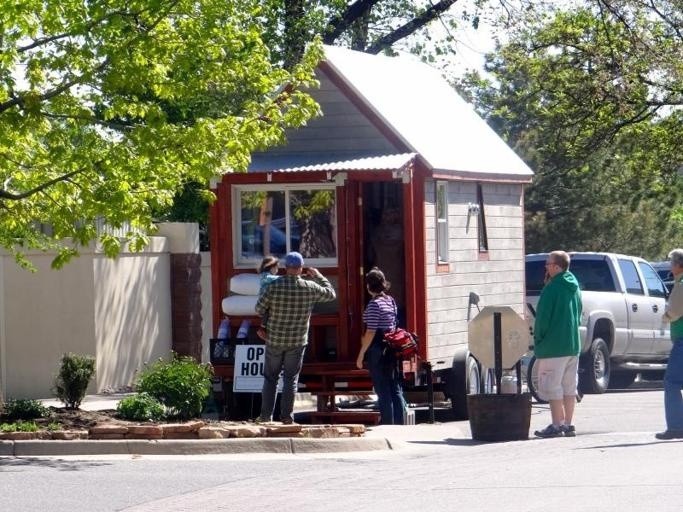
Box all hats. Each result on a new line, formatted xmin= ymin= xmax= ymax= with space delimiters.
xmin=285 ymin=251 xmax=304 ymax=266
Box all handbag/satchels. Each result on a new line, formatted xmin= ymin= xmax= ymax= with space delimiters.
xmin=383 ymin=299 xmax=418 ymax=358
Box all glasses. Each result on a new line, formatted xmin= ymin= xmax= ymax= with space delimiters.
xmin=544 ymin=261 xmax=558 ymax=267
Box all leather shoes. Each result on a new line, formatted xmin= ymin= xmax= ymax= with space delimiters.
xmin=655 ymin=429 xmax=683 ymax=441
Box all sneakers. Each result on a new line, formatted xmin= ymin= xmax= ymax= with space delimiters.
xmin=534 ymin=424 xmax=565 ymax=437
xmin=560 ymin=425 xmax=576 ymax=436
xmin=283 ymin=415 xmax=294 ymax=424
xmin=255 ymin=416 xmax=272 ymax=422
xmin=256 ymin=324 xmax=266 ymax=341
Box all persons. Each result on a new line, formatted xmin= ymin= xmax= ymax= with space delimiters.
xmin=356 ymin=268 xmax=405 ymax=426
xmin=255 ymin=251 xmax=336 ymax=425
xmin=655 ymin=248 xmax=683 ymax=440
xmin=256 ymin=256 xmax=307 ymax=341
xmin=534 ymin=250 xmax=583 ymax=437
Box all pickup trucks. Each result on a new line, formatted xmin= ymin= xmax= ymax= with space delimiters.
xmin=525 ymin=251 xmax=673 ymax=394
